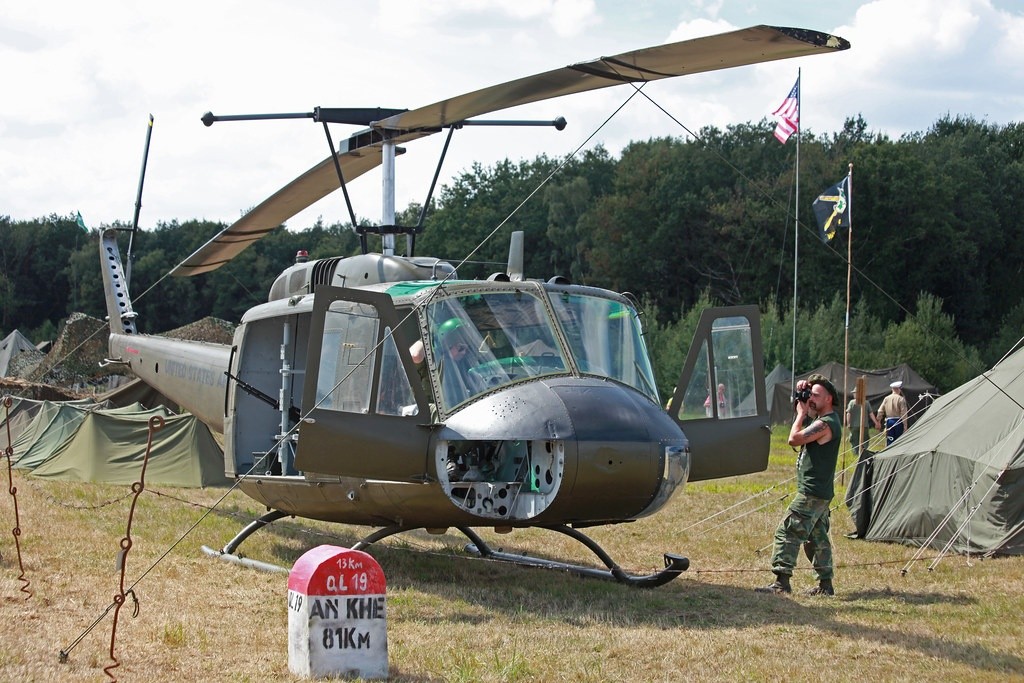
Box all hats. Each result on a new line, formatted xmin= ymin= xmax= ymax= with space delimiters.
xmin=890 ymin=381 xmax=903 ymax=389
xmin=851 ymin=387 xmax=857 ymax=393
xmin=807 ymin=374 xmax=840 ymax=407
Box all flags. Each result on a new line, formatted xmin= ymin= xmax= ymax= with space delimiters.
xmin=812 ymin=177 xmax=849 ymax=246
xmin=771 ymin=76 xmax=798 ymax=145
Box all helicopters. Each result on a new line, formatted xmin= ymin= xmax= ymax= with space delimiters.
xmin=96 ymin=24 xmax=851 ymax=590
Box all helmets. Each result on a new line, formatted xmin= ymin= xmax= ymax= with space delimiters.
xmin=437 ymin=317 xmax=470 ymax=344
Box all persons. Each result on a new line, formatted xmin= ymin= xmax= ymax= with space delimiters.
xmin=755 ymin=374 xmax=842 ymax=596
xmin=666 ymin=386 xmax=685 ymax=420
xmin=397 ymin=317 xmax=479 ymax=415
xmin=846 ymin=380 xmax=908 ymax=456
xmin=703 ymin=382 xmax=726 ymax=419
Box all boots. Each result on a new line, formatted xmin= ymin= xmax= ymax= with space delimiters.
xmin=811 ymin=579 xmax=834 ymax=595
xmin=754 ymin=574 xmax=791 ymax=593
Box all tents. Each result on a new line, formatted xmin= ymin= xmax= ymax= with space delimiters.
xmin=769 ymin=361 xmax=936 ymax=429
xmin=1 ymin=312 xmax=238 ymax=490
xmin=732 ymin=363 xmax=797 ymax=428
xmin=844 ymin=349 xmax=1024 ymax=557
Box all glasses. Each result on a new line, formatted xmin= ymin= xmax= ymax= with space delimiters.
xmin=456 ymin=345 xmax=469 ymax=352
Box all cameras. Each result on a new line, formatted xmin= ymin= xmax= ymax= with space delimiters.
xmin=794 ymin=385 xmax=810 ymax=403
